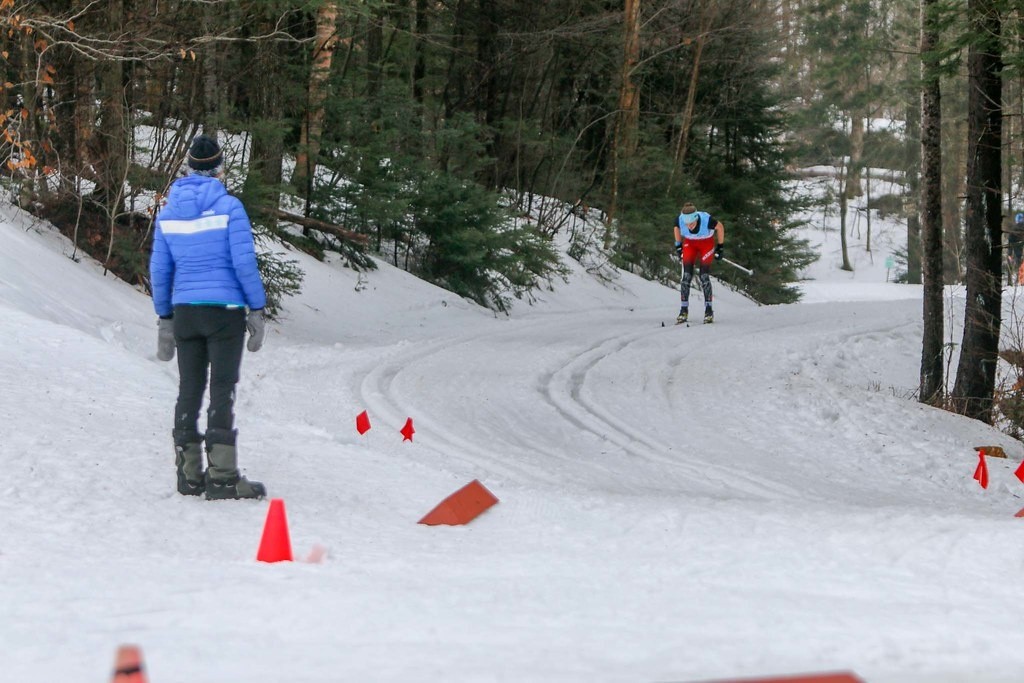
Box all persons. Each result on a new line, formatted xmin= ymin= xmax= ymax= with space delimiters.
xmin=1007 ymin=212 xmax=1024 ymax=286
xmin=147 ymin=137 xmax=269 ymax=501
xmin=672 ymin=202 xmax=725 ymax=325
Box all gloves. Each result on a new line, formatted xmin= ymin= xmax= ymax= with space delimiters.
xmin=246 ymin=310 xmax=264 ymax=352
xmin=157 ymin=313 xmax=175 ymax=361
xmin=675 ymin=242 xmax=683 ymax=259
xmin=714 ymin=243 xmax=723 ymax=260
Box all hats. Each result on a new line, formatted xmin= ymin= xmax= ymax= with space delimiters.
xmin=187 ymin=136 xmax=223 ymax=171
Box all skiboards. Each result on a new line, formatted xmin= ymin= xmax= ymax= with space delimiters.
xmin=661 ymin=318 xmax=709 ymax=327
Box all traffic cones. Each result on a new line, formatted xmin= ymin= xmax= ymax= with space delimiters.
xmin=255 ymin=498 xmax=294 ymax=563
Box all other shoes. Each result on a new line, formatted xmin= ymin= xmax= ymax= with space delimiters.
xmin=677 ymin=309 xmax=688 ymax=323
xmin=703 ymin=310 xmax=713 ymax=324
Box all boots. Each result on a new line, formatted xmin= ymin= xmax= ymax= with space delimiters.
xmin=203 ymin=428 xmax=265 ymax=500
xmin=172 ymin=428 xmax=206 ymax=496
